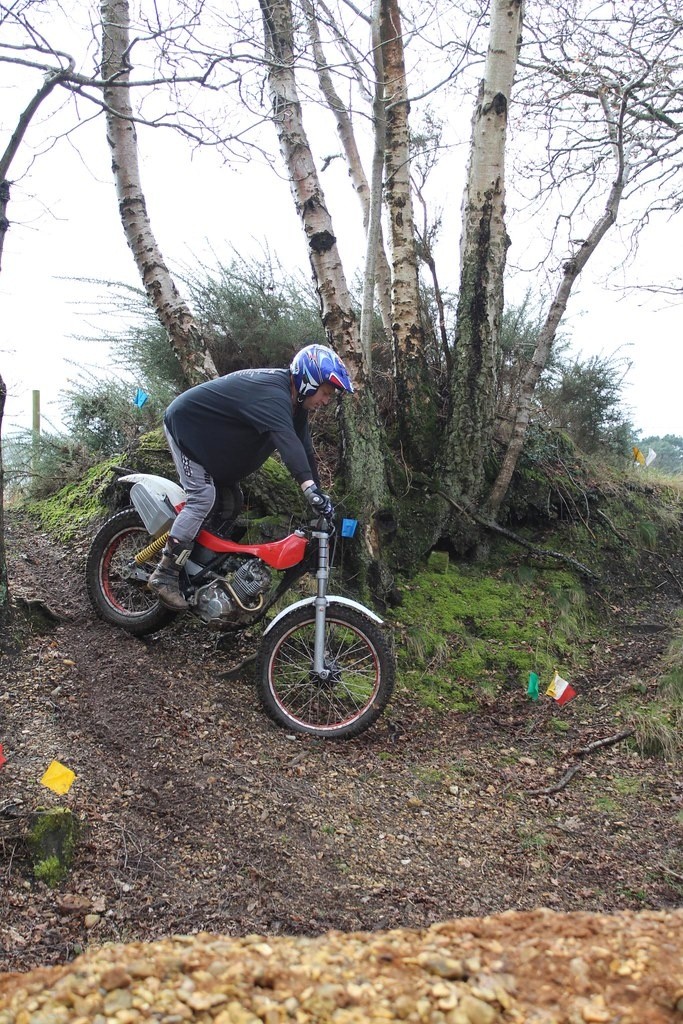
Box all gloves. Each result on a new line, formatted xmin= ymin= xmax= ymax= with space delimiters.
xmin=303 ymin=482 xmax=334 ymax=519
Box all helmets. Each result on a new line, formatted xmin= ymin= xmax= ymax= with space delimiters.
xmin=289 ymin=343 xmax=355 ymax=397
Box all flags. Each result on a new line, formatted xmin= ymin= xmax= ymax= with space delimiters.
xmin=342 ymin=519 xmax=357 ymax=537
xmin=134 ymin=388 xmax=147 ymax=409
xmin=633 ymin=447 xmax=646 ymax=465
xmin=546 ymin=675 xmax=576 ymax=707
xmin=645 ymin=448 xmax=656 ymax=467
xmin=41 ymin=759 xmax=75 ymax=795
xmin=528 ymin=673 xmax=538 ymax=700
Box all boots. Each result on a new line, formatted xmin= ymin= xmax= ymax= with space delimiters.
xmin=147 ymin=536 xmax=190 ymax=610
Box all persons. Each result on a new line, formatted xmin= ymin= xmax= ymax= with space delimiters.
xmin=147 ymin=344 xmax=354 ymax=610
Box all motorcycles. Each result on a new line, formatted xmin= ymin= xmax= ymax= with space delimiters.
xmin=82 ymin=465 xmax=395 ymax=747
